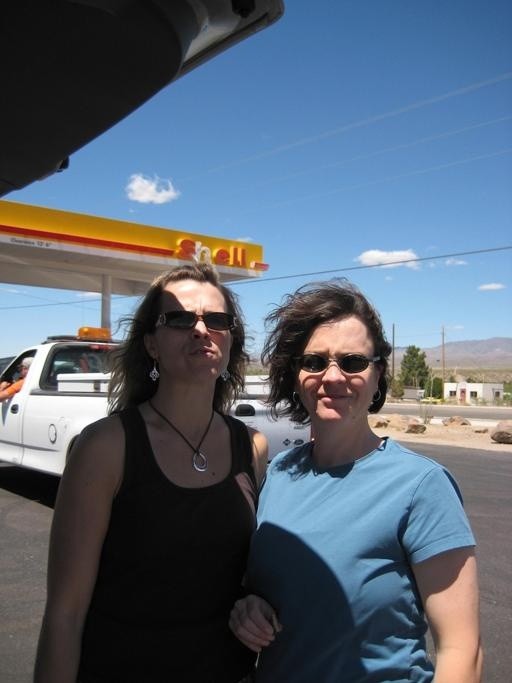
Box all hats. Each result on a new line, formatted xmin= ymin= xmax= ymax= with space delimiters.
xmin=15 ymin=357 xmax=35 ymax=368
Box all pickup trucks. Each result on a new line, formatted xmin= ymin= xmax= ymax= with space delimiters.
xmin=0 ymin=324 xmax=312 ymax=480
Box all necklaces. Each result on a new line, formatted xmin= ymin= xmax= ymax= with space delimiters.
xmin=146 ymin=398 xmax=214 ymax=472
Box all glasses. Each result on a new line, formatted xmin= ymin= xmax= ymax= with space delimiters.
xmin=152 ymin=309 xmax=239 ymax=334
xmin=294 ymin=352 xmax=382 ymax=374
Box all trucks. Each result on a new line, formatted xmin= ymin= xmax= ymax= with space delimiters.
xmin=422 ymin=395 xmax=444 ymax=405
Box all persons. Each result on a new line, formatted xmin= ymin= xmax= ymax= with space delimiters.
xmin=0 ymin=355 xmax=37 ymax=403
xmin=229 ymin=278 xmax=483 ymax=683
xmin=33 ymin=255 xmax=268 ymax=682
